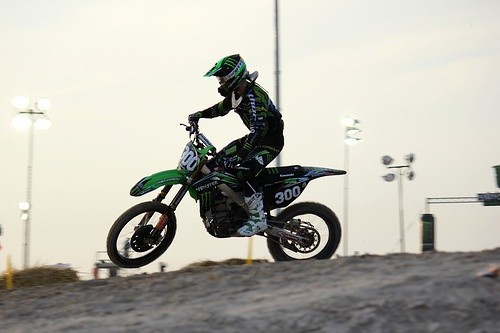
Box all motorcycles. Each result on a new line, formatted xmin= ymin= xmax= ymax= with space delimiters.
xmin=105 ymin=116 xmax=351 ymax=269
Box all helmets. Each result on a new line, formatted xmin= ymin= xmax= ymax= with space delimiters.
xmin=202 ymin=54 xmax=247 ymax=95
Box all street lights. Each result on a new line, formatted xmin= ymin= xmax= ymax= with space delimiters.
xmin=340 ymin=119 xmax=362 ymax=258
xmin=381 ymin=152 xmax=415 ymax=253
xmin=19 ymin=99 xmax=44 ymax=272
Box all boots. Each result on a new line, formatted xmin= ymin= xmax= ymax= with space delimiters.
xmin=237 ymin=193 xmax=267 ymax=236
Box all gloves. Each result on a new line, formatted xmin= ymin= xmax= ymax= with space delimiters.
xmin=224 ymin=153 xmax=243 ymax=169
xmin=188 ymin=111 xmax=203 ymax=126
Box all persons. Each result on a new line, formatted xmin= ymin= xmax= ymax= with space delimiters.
xmin=188 ymin=53 xmax=286 ymax=238
